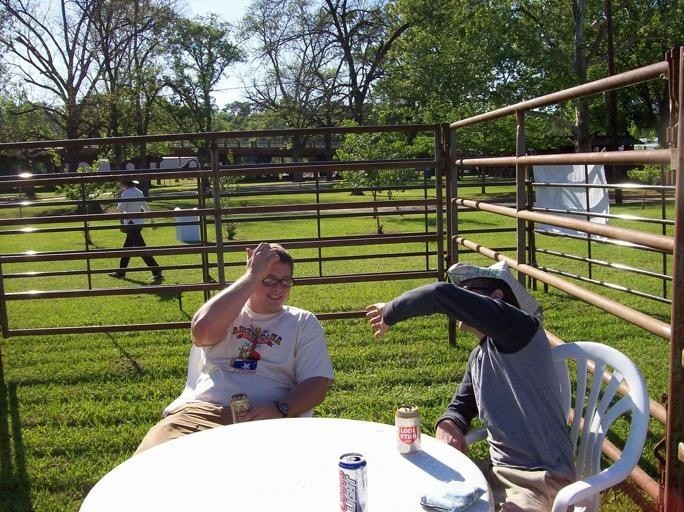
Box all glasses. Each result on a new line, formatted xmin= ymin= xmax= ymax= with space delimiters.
xmin=263 ymin=276 xmax=294 ymax=289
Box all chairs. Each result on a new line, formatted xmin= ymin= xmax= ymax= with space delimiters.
xmin=452 ymin=341 xmax=650 ymax=511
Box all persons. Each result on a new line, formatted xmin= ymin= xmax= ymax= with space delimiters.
xmin=365 ymin=260 xmax=577 ymax=512
xmin=131 ymin=242 xmax=337 ymax=456
xmin=108 ymin=178 xmax=163 ymax=285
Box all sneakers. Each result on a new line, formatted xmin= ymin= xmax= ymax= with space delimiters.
xmin=147 ymin=276 xmax=165 ymax=281
xmin=109 ymin=272 xmax=122 ymax=279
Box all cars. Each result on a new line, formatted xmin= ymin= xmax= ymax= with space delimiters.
xmin=629 ymin=136 xmax=661 ymax=150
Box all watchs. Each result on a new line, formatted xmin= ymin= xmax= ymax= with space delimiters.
xmin=272 ymin=400 xmax=290 ymax=418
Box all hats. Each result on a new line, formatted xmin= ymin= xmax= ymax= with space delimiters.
xmin=447 ymin=260 xmax=543 ymax=319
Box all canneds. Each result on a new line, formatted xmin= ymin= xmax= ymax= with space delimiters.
xmin=229 ymin=392 xmax=249 ymax=423
xmin=338 ymin=452 xmax=369 ymax=512
xmin=395 ymin=404 xmax=421 ymax=454
